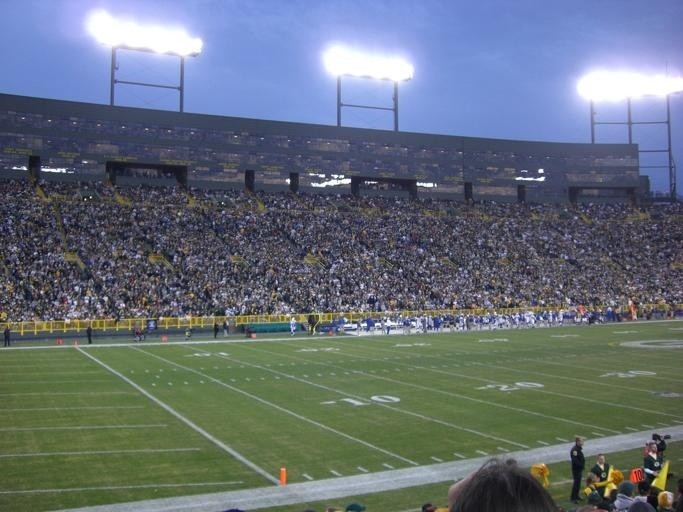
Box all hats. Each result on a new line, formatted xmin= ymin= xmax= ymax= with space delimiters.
xmin=620 ymin=481 xmax=674 ymax=509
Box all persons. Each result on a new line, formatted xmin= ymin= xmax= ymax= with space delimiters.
xmin=422 ymin=458 xmax=566 ymax=512
xmin=0 ymin=179 xmax=682 ymax=348
xmin=570 ymin=430 xmax=683 ymax=512
